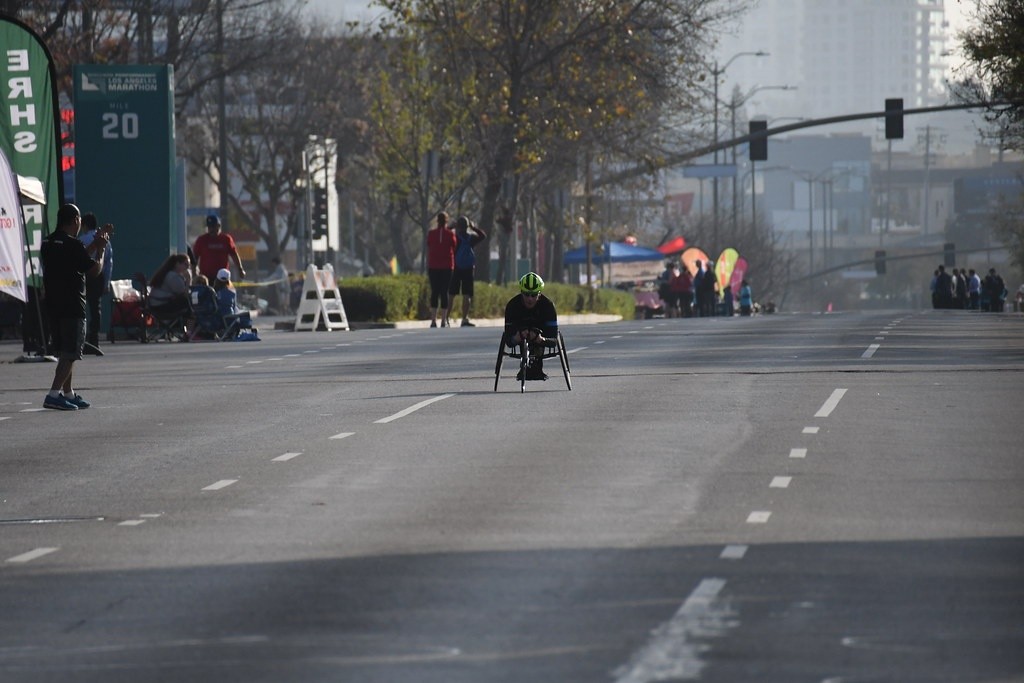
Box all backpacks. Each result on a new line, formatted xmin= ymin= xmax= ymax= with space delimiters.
xmin=454 ymin=233 xmax=477 ymax=274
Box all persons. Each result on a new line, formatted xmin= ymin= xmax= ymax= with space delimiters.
xmin=427 ymin=212 xmax=488 ymax=328
xmin=659 ymin=259 xmax=752 ymax=317
xmin=40 ymin=202 xmax=291 ymax=412
xmin=929 ymin=265 xmax=1024 ymax=313
xmin=505 ymin=272 xmax=559 ymax=380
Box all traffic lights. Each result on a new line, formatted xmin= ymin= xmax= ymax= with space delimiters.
xmin=310 ymin=182 xmax=329 ymax=241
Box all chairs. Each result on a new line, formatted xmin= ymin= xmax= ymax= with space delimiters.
xmin=189 ymin=284 xmax=252 ymax=344
xmin=140 ymin=285 xmax=184 ymax=343
xmin=106 ymin=279 xmax=148 ymax=343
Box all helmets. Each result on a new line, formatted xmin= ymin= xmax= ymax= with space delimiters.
xmin=518 ymin=272 xmax=545 ymax=294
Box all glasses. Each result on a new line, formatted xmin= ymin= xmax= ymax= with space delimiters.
xmin=523 ymin=291 xmax=538 ymax=297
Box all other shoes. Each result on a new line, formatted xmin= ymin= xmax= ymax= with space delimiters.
xmin=183 ymin=332 xmax=203 ymax=341
xmin=43 ymin=392 xmax=78 ymax=409
xmin=440 ymin=320 xmax=446 ymax=327
xmin=430 ymin=320 xmax=437 ymax=328
xmin=64 ymin=393 xmax=91 ymax=408
xmin=461 ymin=320 xmax=475 ymax=328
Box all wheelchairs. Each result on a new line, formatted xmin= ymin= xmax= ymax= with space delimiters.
xmin=494 ymin=329 xmax=572 ymax=394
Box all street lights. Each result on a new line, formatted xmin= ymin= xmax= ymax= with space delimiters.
xmin=712 ymin=50 xmax=812 ymax=259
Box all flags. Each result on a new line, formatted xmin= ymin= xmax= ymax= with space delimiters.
xmin=0 ymin=16 xmax=64 ymax=302
xmin=681 ymin=246 xmax=748 ymax=301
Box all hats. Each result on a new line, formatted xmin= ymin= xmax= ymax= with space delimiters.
xmin=206 ymin=214 xmax=221 ymax=227
xmin=457 ymin=215 xmax=470 ymax=228
xmin=216 ymin=268 xmax=231 ymax=282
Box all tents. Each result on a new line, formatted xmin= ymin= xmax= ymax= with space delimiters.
xmin=564 ymin=241 xmax=666 ymax=289
